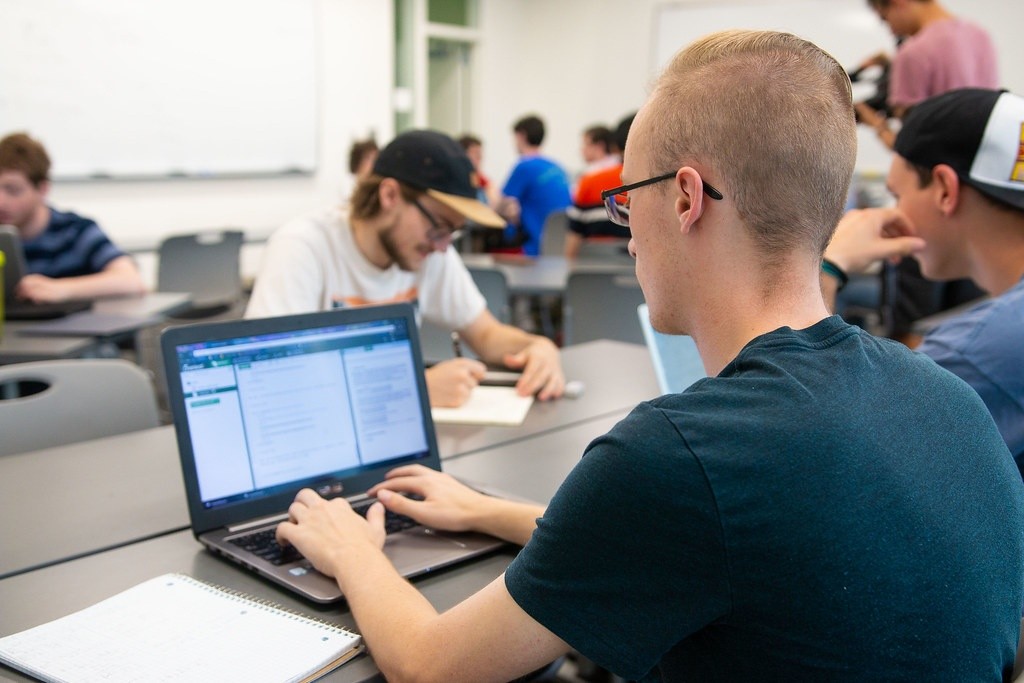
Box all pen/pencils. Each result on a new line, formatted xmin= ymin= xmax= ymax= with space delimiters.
xmin=451 ymin=332 xmax=461 ymax=357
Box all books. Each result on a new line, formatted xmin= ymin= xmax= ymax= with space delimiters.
xmin=0 ymin=571 xmax=365 ymax=683
xmin=430 ymin=371 xmax=535 ymax=425
xmin=638 ymin=304 xmax=708 ymax=396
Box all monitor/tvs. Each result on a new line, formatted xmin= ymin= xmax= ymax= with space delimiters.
xmin=636 ymin=303 xmax=709 ymax=396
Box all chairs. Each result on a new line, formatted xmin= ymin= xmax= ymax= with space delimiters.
xmin=158 ymin=231 xmax=245 ymax=320
xmin=421 ymin=266 xmax=508 ymax=367
xmin=562 ymin=271 xmax=647 ymax=346
xmin=0 ymin=358 xmax=163 ymax=458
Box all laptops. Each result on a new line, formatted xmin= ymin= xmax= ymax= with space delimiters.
xmin=157 ymin=302 xmax=510 ymax=607
xmin=0 ymin=226 xmax=94 ymax=319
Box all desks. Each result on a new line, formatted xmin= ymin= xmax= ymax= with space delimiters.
xmin=0 ymin=293 xmax=193 ymax=369
xmin=460 ymin=258 xmax=639 ymax=343
xmin=0 ymin=339 xmax=662 ymax=683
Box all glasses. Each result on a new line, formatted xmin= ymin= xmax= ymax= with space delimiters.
xmin=601 ymin=171 xmax=723 ymax=226
xmin=402 ymin=191 xmax=471 ymax=244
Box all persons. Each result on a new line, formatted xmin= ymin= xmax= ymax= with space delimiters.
xmin=242 ymin=130 xmax=564 ymax=408
xmin=0 ymin=133 xmax=140 ymax=306
xmin=853 ymin=0 xmax=999 ymax=151
xmin=275 ymin=31 xmax=1024 ymax=683
xmin=350 ymin=113 xmax=650 ymax=332
xmin=818 ymin=86 xmax=1024 ymax=481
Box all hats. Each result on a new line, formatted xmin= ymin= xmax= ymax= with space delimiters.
xmin=893 ymin=86 xmax=1024 ymax=211
xmin=372 ymin=130 xmax=507 ymax=229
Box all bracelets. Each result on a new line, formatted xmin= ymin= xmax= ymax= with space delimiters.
xmin=821 ymin=258 xmax=848 ymax=291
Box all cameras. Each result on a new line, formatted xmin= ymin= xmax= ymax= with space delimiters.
xmin=847 ymin=55 xmax=893 ymax=125
